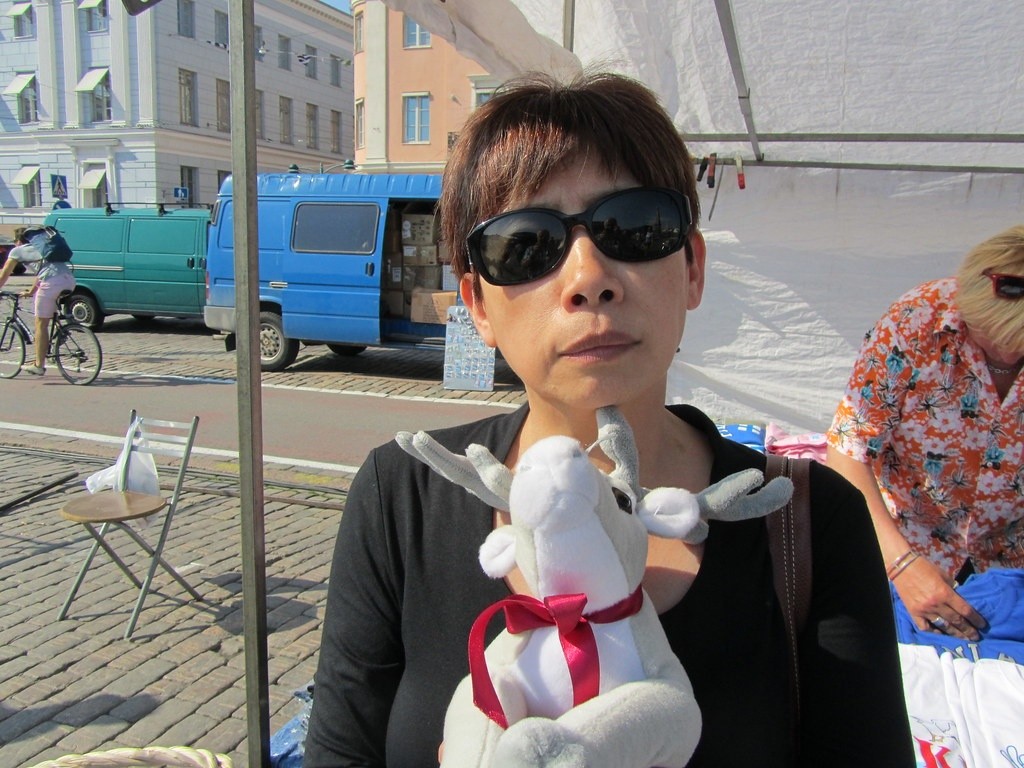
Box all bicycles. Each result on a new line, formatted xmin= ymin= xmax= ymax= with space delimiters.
xmin=0 ymin=289 xmax=103 ymax=386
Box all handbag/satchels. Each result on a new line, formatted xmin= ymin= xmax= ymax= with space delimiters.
xmin=83 ymin=415 xmax=162 ymax=530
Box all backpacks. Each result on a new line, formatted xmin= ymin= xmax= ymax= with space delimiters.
xmin=22 ymin=223 xmax=75 ymax=275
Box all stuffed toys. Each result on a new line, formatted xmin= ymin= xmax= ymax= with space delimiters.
xmin=393 ymin=406 xmax=794 ymax=768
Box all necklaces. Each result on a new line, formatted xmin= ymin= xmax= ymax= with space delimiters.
xmin=986 ymin=364 xmax=1021 ymax=375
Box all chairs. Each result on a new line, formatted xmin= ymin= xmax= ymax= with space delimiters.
xmin=56 ymin=410 xmax=204 ymax=639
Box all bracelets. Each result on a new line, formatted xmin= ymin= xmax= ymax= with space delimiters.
xmin=885 ymin=547 xmax=920 ymax=584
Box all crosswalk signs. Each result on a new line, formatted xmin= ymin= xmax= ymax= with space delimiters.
xmin=50 ymin=173 xmax=68 ymax=198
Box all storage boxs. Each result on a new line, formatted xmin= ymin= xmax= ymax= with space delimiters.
xmin=381 ymin=209 xmax=460 ymax=325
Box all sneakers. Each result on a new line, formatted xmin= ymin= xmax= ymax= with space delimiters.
xmin=58 ymin=326 xmax=68 ymax=346
xmin=20 ymin=363 xmax=46 ymax=376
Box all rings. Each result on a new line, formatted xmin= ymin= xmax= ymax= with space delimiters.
xmin=931 ymin=618 xmax=943 ymax=627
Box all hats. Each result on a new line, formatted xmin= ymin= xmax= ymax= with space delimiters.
xmin=13 ymin=227 xmax=28 ymax=243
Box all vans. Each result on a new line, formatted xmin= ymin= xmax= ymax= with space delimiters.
xmin=41 ymin=203 xmax=218 ymax=332
xmin=204 ymin=167 xmax=504 ymax=373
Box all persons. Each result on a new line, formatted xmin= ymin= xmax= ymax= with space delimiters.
xmin=812 ymin=225 xmax=1024 ymax=655
xmin=303 ymin=69 xmax=915 ymax=768
xmin=0 ymin=225 xmax=78 ymax=376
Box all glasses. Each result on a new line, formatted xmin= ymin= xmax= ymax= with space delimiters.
xmin=466 ymin=185 xmax=693 ymax=286
xmin=983 ymin=269 xmax=1024 ymax=301
